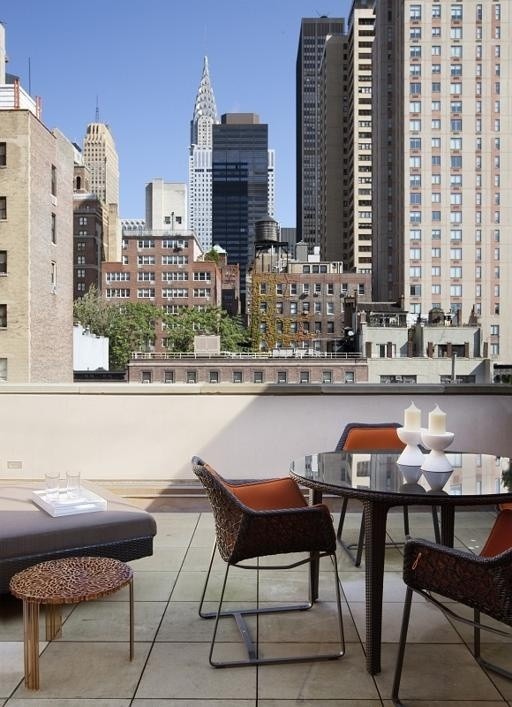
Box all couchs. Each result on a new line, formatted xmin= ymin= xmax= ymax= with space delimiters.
xmin=1 ymin=479 xmax=157 ymax=593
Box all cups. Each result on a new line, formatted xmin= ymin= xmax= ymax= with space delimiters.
xmin=65 ymin=469 xmax=81 ymax=499
xmin=45 ymin=469 xmax=61 ymax=502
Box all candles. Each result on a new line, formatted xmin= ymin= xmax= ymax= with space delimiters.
xmin=427 ymin=401 xmax=447 ymax=435
xmin=401 ymin=399 xmax=422 ymax=429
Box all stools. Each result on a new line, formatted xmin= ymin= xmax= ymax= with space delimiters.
xmin=9 ymin=554 xmax=136 ymax=690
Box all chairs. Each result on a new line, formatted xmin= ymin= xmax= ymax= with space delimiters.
xmin=338 ymin=424 xmax=441 ymax=566
xmin=392 ymin=509 xmax=511 ymax=699
xmin=192 ymin=454 xmax=346 ymax=668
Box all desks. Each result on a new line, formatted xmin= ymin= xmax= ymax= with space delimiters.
xmin=290 ymin=448 xmax=512 ymax=675
